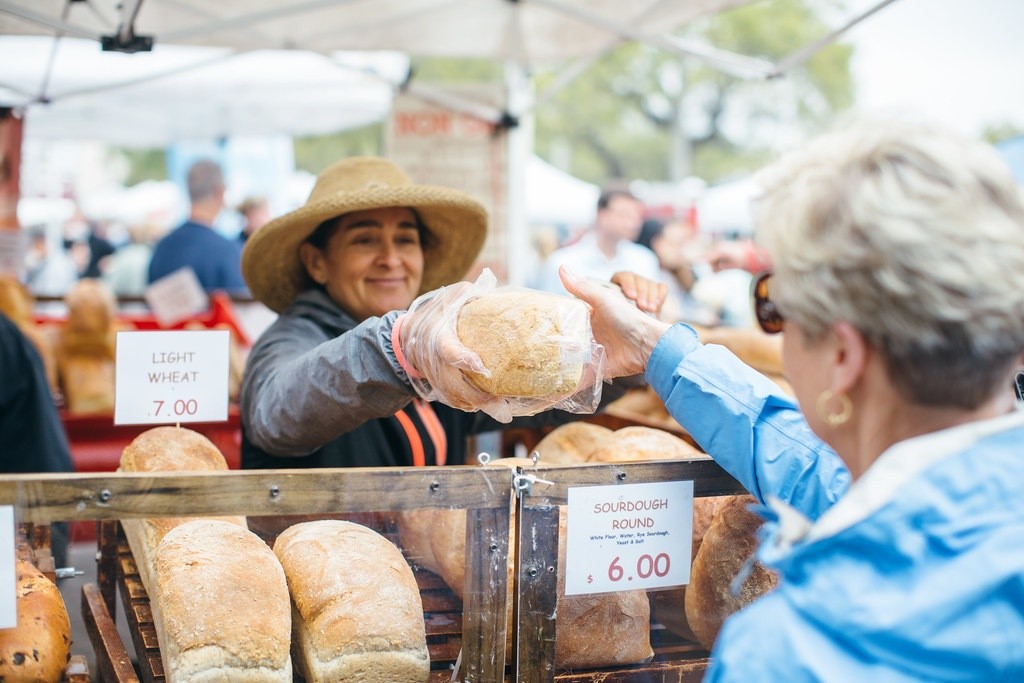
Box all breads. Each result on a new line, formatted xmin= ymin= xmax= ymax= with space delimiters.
xmin=0 ymin=518 xmax=70 ymax=683
xmin=103 ymin=422 xmax=786 ymax=683
xmin=0 ymin=269 xmax=252 ymax=417
xmin=456 ymin=290 xmax=585 ymax=401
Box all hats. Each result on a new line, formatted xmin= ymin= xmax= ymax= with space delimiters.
xmin=241 ymin=157 xmax=488 ymax=313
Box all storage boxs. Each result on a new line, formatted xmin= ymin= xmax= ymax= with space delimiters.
xmin=19 ymin=286 xmax=255 ymax=550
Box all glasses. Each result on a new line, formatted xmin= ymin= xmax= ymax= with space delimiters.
xmin=754 ymin=274 xmax=785 ymax=333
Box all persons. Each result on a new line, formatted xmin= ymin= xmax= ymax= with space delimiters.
xmin=238 ymin=160 xmax=666 ymax=550
xmin=22 ymin=156 xmax=270 ymax=314
xmin=540 ymin=117 xmax=1024 ymax=683
xmin=0 ymin=312 xmax=74 ymax=589
